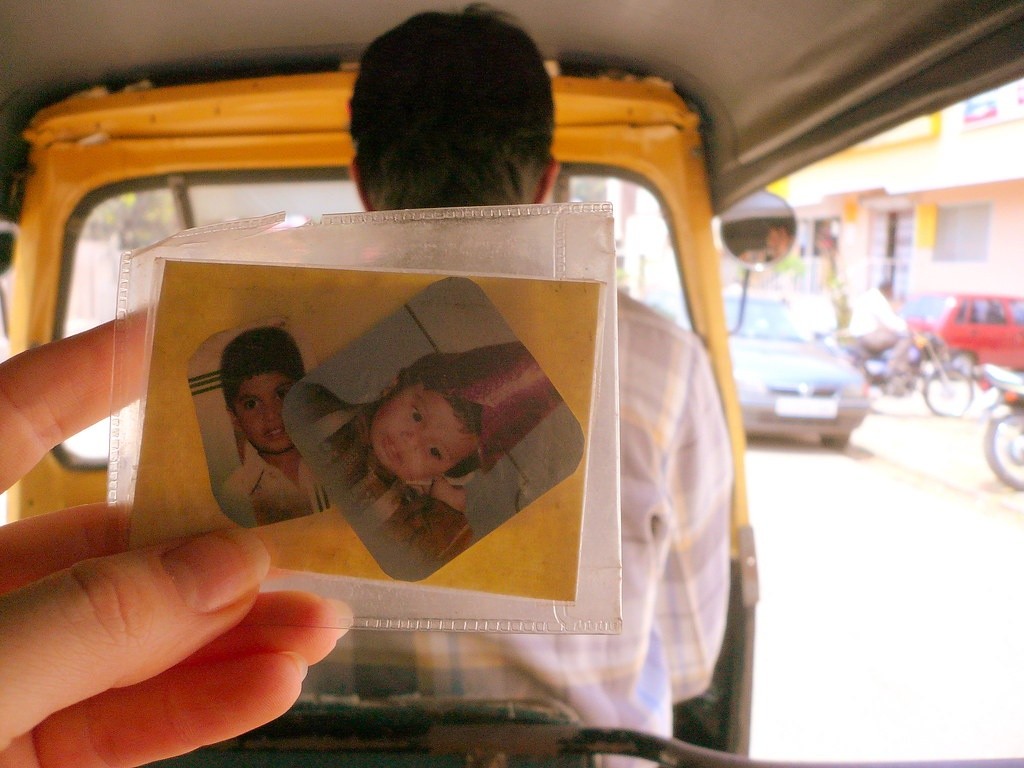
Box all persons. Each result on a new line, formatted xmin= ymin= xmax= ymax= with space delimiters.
xmin=1 ymin=297 xmax=354 ymax=767
xmin=739 ymin=226 xmax=790 ymax=264
xmin=848 ymin=282 xmax=930 ymax=396
xmin=257 ymin=0 xmax=733 ymax=768
xmin=217 ymin=320 xmax=565 ymax=572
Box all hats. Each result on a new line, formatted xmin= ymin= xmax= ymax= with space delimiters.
xmin=440 ymin=339 xmax=563 ymax=477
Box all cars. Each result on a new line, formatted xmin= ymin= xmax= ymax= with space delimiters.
xmin=904 ymin=290 xmax=1024 ymax=374
xmin=720 ymin=292 xmax=870 ymax=453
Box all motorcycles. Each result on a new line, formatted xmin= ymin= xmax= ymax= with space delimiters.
xmin=845 ymin=321 xmax=976 ymax=421
xmin=977 ymin=360 xmax=1024 ymax=495
